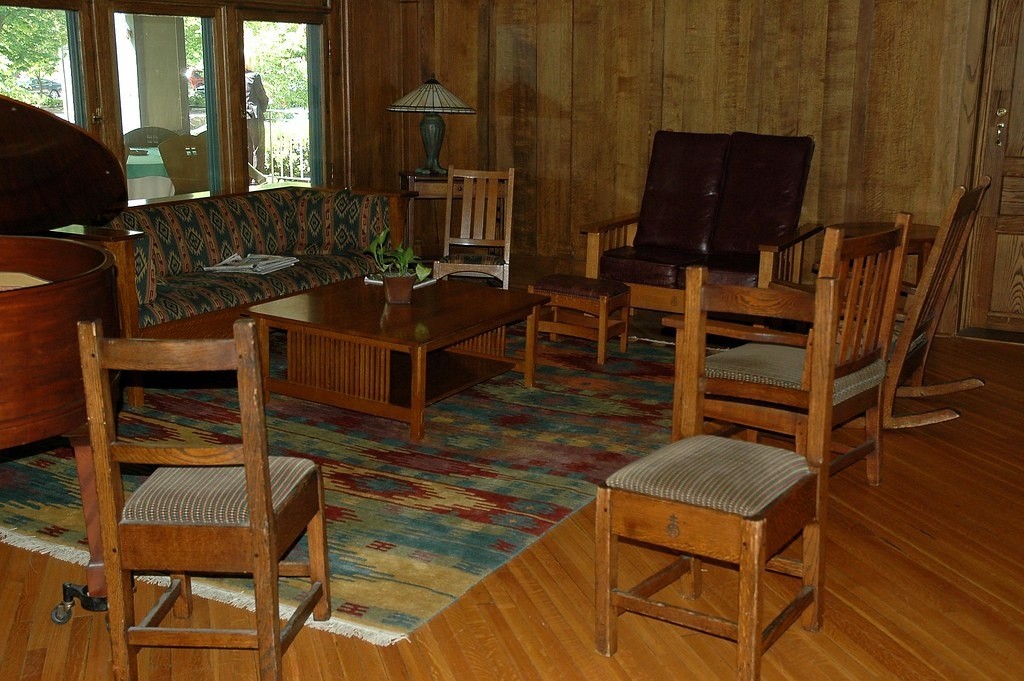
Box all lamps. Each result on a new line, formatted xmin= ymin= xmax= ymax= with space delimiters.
xmin=387 ymin=72 xmax=477 ymax=175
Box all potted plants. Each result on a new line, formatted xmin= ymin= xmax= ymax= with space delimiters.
xmin=369 ymin=231 xmax=431 ymax=307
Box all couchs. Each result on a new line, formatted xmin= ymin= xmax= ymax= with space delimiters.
xmin=50 ymin=186 xmax=419 ymax=408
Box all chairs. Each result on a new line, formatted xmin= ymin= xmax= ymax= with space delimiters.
xmin=123 ymin=125 xmax=179 ymax=148
xmin=76 ymin=318 xmax=330 ymax=681
xmin=660 ymin=211 xmax=911 ymax=521
xmin=434 ymin=165 xmax=516 ymax=291
xmin=754 ymin=175 xmax=994 ymax=429
xmin=159 ymin=128 xmax=210 ymax=193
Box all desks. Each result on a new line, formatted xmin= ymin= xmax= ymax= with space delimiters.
xmin=126 ymin=147 xmax=192 ymax=199
xmin=401 ymin=170 xmax=504 ymax=257
xmin=249 ymin=269 xmax=552 ymax=441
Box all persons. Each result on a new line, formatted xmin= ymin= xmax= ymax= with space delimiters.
xmin=245 ymin=67 xmax=268 ymax=181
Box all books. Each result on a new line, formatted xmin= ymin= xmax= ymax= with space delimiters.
xmin=364 ymin=270 xmax=437 ymax=289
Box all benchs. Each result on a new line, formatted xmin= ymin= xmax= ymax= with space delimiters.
xmin=580 ymin=131 xmax=822 ymax=308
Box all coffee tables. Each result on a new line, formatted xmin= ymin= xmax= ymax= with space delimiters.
xmin=827 ymin=223 xmax=943 ymax=314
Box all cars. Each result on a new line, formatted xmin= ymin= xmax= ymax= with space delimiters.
xmin=21 ymin=78 xmax=61 ymax=99
xmin=189 ymin=69 xmax=204 ymax=90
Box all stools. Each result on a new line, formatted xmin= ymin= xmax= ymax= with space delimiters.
xmin=595 ymin=432 xmax=819 ymax=681
xmin=527 ymin=273 xmax=632 ymax=365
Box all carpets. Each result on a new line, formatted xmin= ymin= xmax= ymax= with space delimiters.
xmin=0 ymin=312 xmax=731 ymax=646
